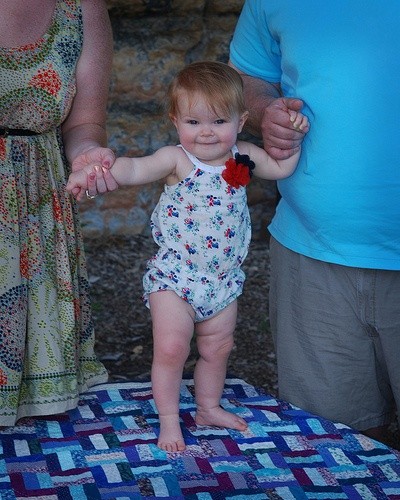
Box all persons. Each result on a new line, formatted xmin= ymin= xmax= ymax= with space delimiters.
xmin=66 ymin=62 xmax=310 ymax=452
xmin=0 ymin=0 xmax=118 ymax=430
xmin=228 ymin=0 xmax=400 ymax=451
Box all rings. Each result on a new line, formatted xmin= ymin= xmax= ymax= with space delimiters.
xmin=86 ymin=190 xmax=97 ymax=199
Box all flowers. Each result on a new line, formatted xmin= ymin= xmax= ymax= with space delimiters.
xmin=219 ymin=158 xmax=251 ymax=188
